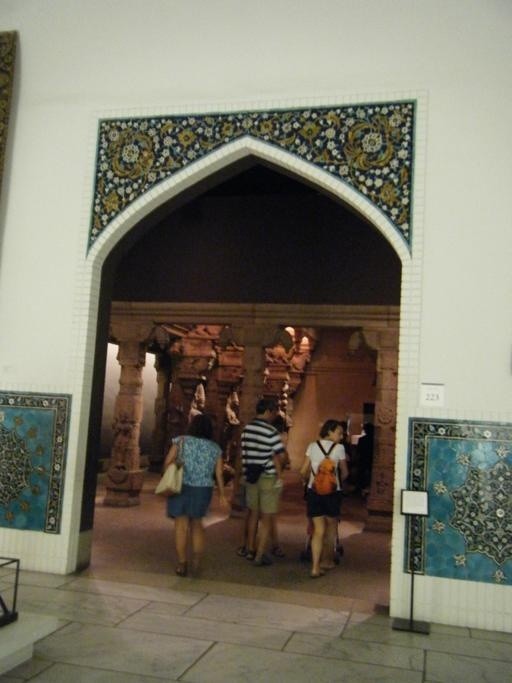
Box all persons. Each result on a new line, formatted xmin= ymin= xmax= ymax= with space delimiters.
xmin=238 ymin=398 xmax=287 ymax=565
xmin=270 ymin=415 xmax=288 ymax=488
xmin=298 ymin=418 xmax=350 ymax=578
xmin=162 ymin=414 xmax=230 ymax=576
xmin=238 ymin=507 xmax=285 ymax=558
xmin=356 ymin=422 xmax=374 ymax=489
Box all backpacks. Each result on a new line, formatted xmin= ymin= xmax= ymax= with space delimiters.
xmin=312 ymin=456 xmax=338 ymax=497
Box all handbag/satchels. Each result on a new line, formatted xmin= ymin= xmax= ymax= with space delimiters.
xmin=246 ymin=463 xmax=263 ymax=485
xmin=155 ymin=463 xmax=184 ymax=496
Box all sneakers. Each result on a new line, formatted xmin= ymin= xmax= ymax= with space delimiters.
xmin=236 ymin=545 xmax=284 ymax=565
xmin=176 ymin=562 xmax=188 ymax=577
